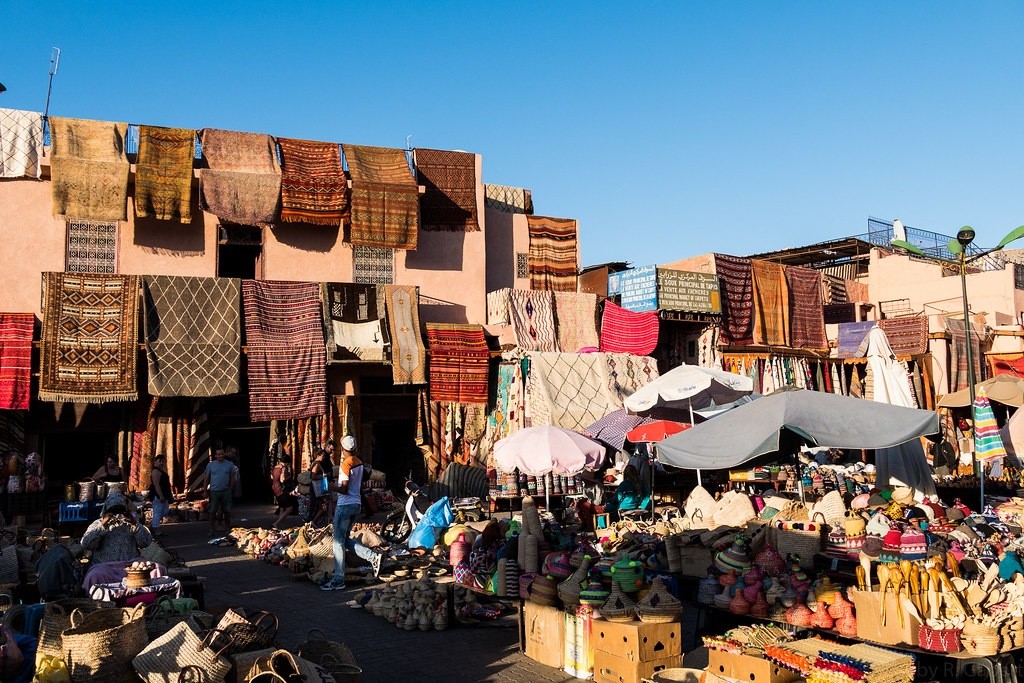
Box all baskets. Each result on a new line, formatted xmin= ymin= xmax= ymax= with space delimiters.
xmin=36 ymin=600 xmax=364 ymax=681
xmin=307 ymin=523 xmax=334 ymax=558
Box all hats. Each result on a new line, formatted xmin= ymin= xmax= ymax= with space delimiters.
xmin=341 ymin=435 xmax=357 ymax=451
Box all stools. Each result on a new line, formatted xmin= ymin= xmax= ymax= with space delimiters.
xmin=24 ymin=602 xmax=59 ymax=638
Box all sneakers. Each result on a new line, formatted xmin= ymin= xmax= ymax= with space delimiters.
xmin=320 ymin=578 xmax=346 ymax=591
xmin=370 ymin=552 xmax=382 ymax=577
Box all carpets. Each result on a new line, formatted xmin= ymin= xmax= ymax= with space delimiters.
xmin=0 ymin=110 xmax=1024 ymax=496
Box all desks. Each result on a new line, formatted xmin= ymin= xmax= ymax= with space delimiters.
xmin=486 ymin=491 xmax=583 ymax=520
xmin=447 ymin=580 xmax=525 ymax=654
xmin=729 ymin=480 xmax=787 ymax=493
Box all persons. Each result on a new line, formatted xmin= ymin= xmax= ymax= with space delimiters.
xmin=302 ymin=440 xmax=335 ymax=528
xmin=80 ymin=492 xmax=183 ymax=606
xmin=91 ymin=453 xmax=126 ymax=485
xmin=270 ymin=453 xmax=295 ymax=528
xmin=320 ymin=435 xmax=383 ymax=590
xmin=582 ymin=440 xmax=685 ymax=522
xmin=150 ymin=454 xmax=174 ymax=537
xmin=350 ymin=450 xmax=375 ymax=520
xmin=205 ymin=444 xmax=241 ymax=538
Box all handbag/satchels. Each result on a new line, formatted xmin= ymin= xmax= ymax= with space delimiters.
xmin=310 ymin=476 xmax=333 ymax=497
xmin=661 ymin=488 xmax=1024 ymax=577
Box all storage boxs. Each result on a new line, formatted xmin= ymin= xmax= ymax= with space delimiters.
xmin=58 ymin=502 xmax=89 ymax=523
xmin=680 ymin=546 xmax=715 ymax=579
xmin=729 ymin=469 xmax=755 ymax=480
xmin=853 ymin=582 xmax=943 ymax=647
xmin=522 ymin=599 xmax=800 ymax=683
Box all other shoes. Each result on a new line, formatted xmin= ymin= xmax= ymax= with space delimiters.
xmin=217 ymin=525 xmax=226 ymax=530
xmin=152 ymin=530 xmax=170 ymax=537
xmin=207 ymin=528 xmax=215 ymax=538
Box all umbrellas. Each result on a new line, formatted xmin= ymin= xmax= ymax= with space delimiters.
xmin=694 ymin=391 xmax=766 ymax=420
xmin=623 ymin=361 xmax=754 ymax=489
xmin=494 ymin=425 xmax=607 ymax=514
xmin=627 ymin=421 xmax=692 ymax=445
xmin=935 ymin=373 xmax=1024 ymax=409
xmin=585 ymin=405 xmax=709 ymax=458
xmin=867 ymin=325 xmax=940 ymax=505
xmin=973 ymin=386 xmax=1008 ymax=512
xmin=654 ymin=384 xmax=940 ymax=504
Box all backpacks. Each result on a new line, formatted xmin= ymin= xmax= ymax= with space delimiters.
xmin=296 ymin=469 xmax=313 ymax=492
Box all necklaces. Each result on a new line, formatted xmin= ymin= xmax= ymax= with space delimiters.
xmin=112 ymin=469 xmax=118 ymax=474
